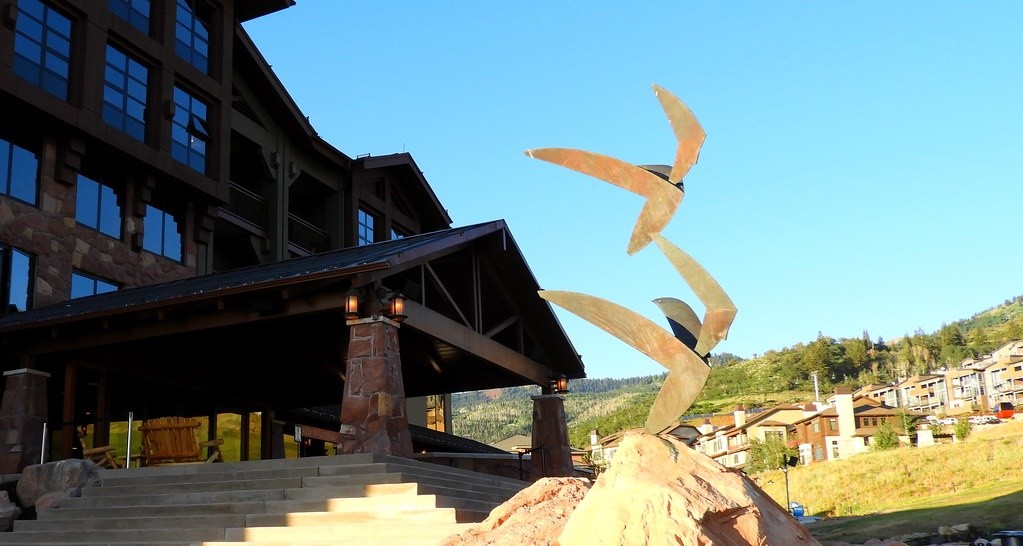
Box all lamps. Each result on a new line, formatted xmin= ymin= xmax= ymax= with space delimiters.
xmin=387 ymin=288 xmax=408 ymax=323
xmin=551 ymin=371 xmax=570 ymax=394
xmin=344 ymin=283 xmax=362 ymax=319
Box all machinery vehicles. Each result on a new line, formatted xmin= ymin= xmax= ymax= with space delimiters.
xmin=790 ymin=503 xmax=825 ymax=524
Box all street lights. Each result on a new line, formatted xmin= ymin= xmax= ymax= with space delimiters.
xmin=811 ymin=371 xmax=820 ymax=401
xmin=782 ymin=453 xmax=798 ymax=514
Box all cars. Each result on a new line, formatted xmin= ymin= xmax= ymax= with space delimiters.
xmin=983 ymin=415 xmax=1000 ymax=424
xmin=939 ymin=417 xmax=958 ymax=425
xmin=968 ymin=416 xmax=986 ymax=425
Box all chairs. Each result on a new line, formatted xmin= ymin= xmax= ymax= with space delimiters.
xmin=138 ymin=416 xmax=224 ymax=467
xmin=70 ymin=429 xmax=120 ymax=471
